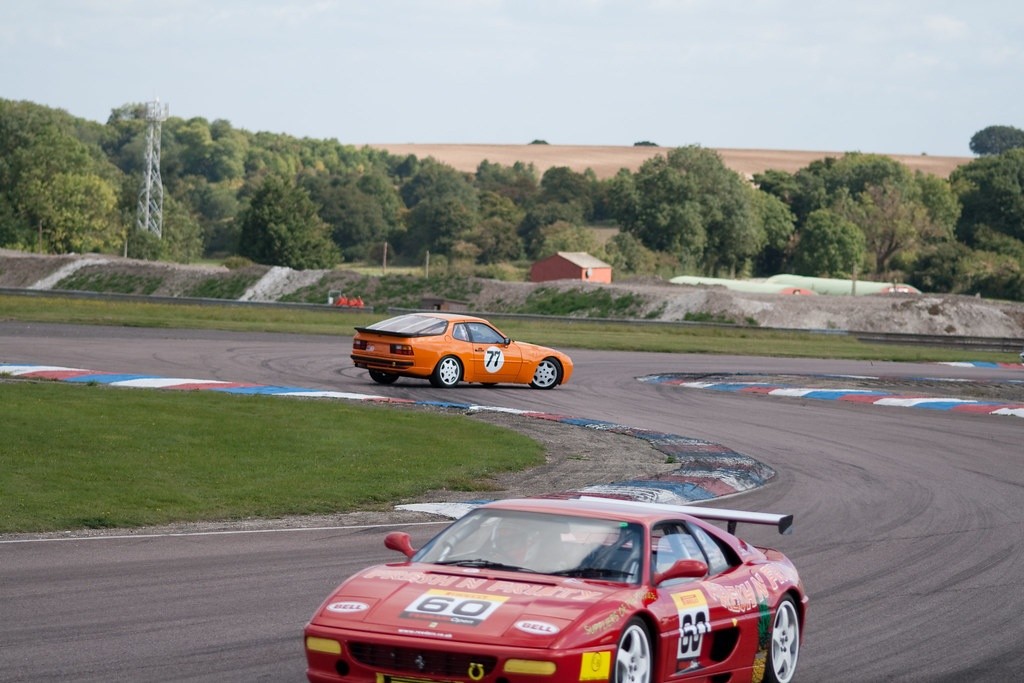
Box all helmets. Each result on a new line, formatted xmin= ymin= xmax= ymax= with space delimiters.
xmin=492 ymin=515 xmax=542 ymax=566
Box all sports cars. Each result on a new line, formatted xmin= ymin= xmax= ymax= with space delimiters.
xmin=303 ymin=493 xmax=810 ymax=683
xmin=349 ymin=312 xmax=575 ymax=390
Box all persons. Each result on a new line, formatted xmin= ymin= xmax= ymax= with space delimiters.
xmin=336 ymin=294 xmax=364 ymax=306
xmin=491 ymin=522 xmax=530 ymax=566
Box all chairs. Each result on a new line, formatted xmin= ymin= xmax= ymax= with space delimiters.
xmin=578 ymin=546 xmax=671 ymax=586
